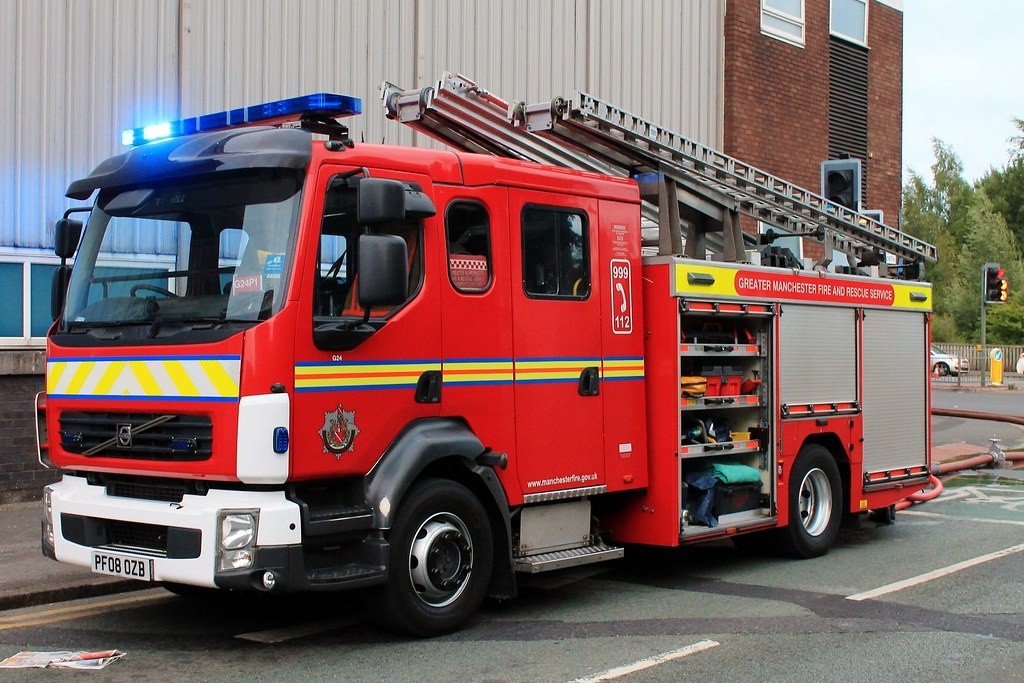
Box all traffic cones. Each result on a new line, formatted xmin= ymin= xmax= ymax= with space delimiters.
xmin=932 ymin=364 xmax=939 ymax=377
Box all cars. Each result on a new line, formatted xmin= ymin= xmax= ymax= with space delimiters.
xmin=930 ymin=344 xmax=970 ymax=377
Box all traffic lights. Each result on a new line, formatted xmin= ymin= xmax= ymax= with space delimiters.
xmin=984 ymin=263 xmax=1004 ymax=302
xmin=858 ymin=210 xmax=883 ymax=232
xmin=999 ymin=277 xmax=1009 ymax=302
xmin=821 ymin=159 xmax=861 ymax=216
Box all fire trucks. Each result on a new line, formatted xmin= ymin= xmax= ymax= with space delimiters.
xmin=33 ymin=72 xmax=945 ymax=642
